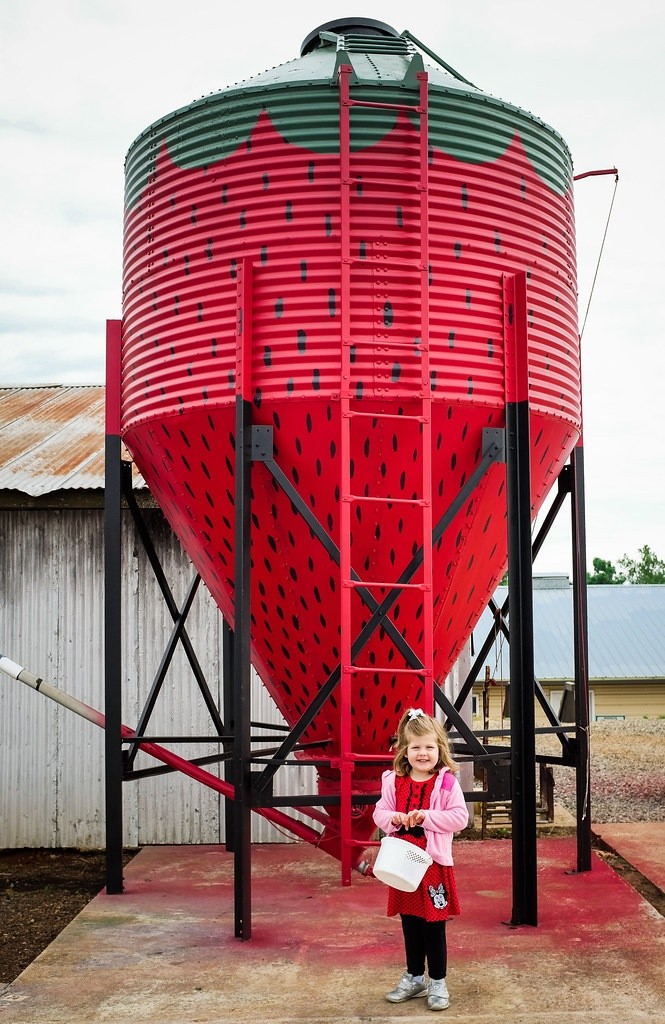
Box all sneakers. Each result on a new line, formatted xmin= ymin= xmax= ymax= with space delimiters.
xmin=428 ymin=978 xmax=449 ymax=1010
xmin=385 ymin=972 xmax=427 ymax=1002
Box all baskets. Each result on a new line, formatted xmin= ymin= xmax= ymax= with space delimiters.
xmin=373 ymin=822 xmax=434 ymax=892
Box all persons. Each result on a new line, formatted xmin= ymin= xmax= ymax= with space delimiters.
xmin=372 ymin=708 xmax=470 ymax=1010
